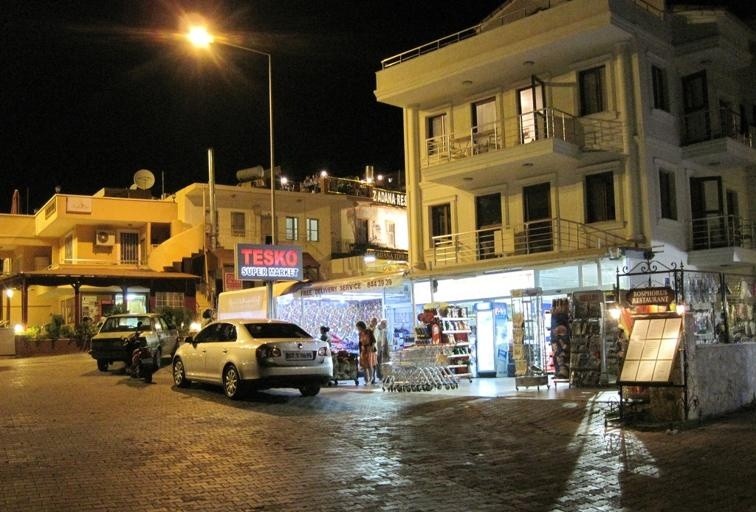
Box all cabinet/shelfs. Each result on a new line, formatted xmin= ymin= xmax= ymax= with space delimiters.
xmin=511 ymin=287 xmax=629 ymax=391
xmin=417 ymin=308 xmax=473 ymax=383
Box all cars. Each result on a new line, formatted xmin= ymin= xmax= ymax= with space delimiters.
xmin=89 ymin=313 xmax=178 ymax=372
xmin=167 ymin=317 xmax=335 ymax=401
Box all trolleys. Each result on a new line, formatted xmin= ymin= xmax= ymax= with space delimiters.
xmin=328 ymin=350 xmax=359 ymax=387
xmin=379 ymin=344 xmax=459 ymax=392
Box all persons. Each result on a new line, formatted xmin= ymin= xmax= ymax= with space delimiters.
xmin=366 ymin=317 xmax=381 ymax=385
xmin=377 ymin=319 xmax=391 ymax=382
xmin=319 ymin=325 xmax=331 ymax=347
xmin=356 ymin=320 xmax=375 ymax=387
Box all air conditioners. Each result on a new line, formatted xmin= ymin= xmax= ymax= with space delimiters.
xmin=95 ymin=229 xmax=115 ymax=247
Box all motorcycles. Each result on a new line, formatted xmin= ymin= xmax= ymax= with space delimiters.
xmin=119 ymin=321 xmax=155 ymax=384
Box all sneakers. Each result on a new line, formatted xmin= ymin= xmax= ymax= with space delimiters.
xmin=365 ymin=376 xmax=373 ymax=385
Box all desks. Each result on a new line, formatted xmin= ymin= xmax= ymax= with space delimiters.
xmin=454 ymin=129 xmax=497 ymax=155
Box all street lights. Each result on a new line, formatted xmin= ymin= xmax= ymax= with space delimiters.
xmin=177 ymin=24 xmax=277 ymax=246
